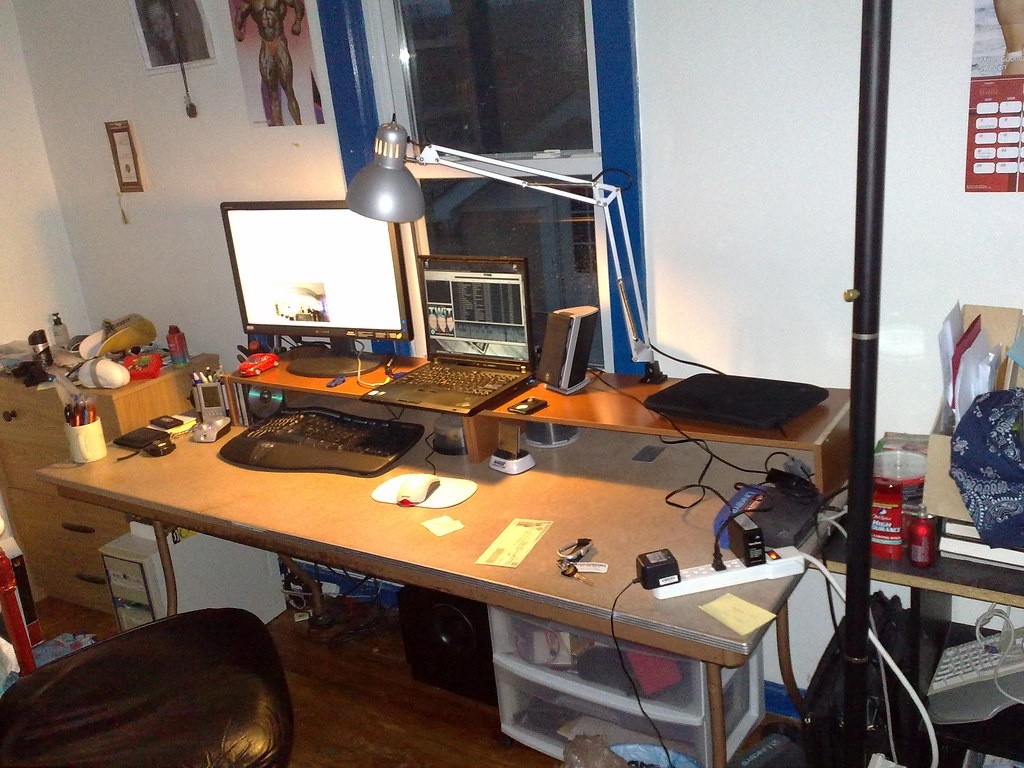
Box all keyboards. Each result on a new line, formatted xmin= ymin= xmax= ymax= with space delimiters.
xmin=927 ymin=626 xmax=1024 ymax=726
xmin=218 ymin=407 xmax=425 ymax=475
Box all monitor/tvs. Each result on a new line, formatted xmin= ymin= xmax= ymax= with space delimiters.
xmin=220 ymin=200 xmax=414 ymax=378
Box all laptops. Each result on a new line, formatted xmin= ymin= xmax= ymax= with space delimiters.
xmin=359 ymin=254 xmax=537 ymax=418
xmin=644 ymin=373 xmax=830 ymax=430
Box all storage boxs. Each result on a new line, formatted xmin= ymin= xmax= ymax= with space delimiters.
xmin=130 ymin=515 xmax=181 ymax=545
xmin=917 ymin=305 xmax=1024 ymax=526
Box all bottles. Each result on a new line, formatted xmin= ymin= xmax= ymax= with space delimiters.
xmin=907 ymin=504 xmax=938 ymax=568
xmin=166 ymin=325 xmax=191 ymax=369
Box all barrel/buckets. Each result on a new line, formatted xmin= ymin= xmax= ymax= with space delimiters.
xmin=871 ymin=449 xmax=927 ymax=561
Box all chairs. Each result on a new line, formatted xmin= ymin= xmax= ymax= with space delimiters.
xmin=0 ymin=605 xmax=298 ymax=768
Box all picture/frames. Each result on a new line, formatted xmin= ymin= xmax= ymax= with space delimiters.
xmin=104 ymin=120 xmax=145 ymax=193
xmin=128 ymin=0 xmax=217 ymax=77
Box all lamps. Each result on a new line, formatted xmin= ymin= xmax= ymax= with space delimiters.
xmin=345 ymin=122 xmax=670 ymax=385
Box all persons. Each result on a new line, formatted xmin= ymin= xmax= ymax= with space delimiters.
xmin=143 ymin=0 xmax=191 ymax=67
xmin=235 ymin=0 xmax=306 ymax=126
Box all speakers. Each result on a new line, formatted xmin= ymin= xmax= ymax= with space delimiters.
xmin=536 ymin=306 xmax=599 ymax=395
xmin=396 ymin=583 xmax=499 ymax=707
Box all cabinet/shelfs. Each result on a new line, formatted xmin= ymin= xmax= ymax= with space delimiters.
xmin=487 ymin=604 xmax=766 ymax=768
xmin=0 ymin=353 xmax=222 ymax=618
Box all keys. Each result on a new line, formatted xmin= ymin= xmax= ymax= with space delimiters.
xmin=561 ymin=564 xmax=593 ymax=586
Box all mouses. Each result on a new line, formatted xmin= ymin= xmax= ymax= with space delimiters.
xmin=397 ymin=473 xmax=440 ymax=504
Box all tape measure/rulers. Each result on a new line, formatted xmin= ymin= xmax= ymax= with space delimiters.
xmin=143 ymin=438 xmax=176 ymax=457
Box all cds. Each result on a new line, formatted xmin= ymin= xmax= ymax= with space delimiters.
xmin=248 ymin=384 xmax=282 ymax=417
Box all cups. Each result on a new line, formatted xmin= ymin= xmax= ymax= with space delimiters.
xmin=64 ymin=415 xmax=108 ymax=463
xmin=28 ymin=330 xmax=53 ymax=370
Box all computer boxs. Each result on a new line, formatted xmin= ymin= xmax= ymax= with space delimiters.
xmin=98 ymin=526 xmax=287 ymax=633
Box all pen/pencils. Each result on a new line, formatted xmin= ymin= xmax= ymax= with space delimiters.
xmin=190 ymin=366 xmax=224 ymax=385
xmin=61 ymin=392 xmax=100 ymax=426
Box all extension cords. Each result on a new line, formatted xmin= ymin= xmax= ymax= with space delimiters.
xmin=651 ymin=546 xmax=804 ymax=599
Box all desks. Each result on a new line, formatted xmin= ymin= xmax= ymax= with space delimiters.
xmin=813 ymin=533 xmax=1024 ymax=768
xmin=30 ymin=352 xmax=849 ymax=768
